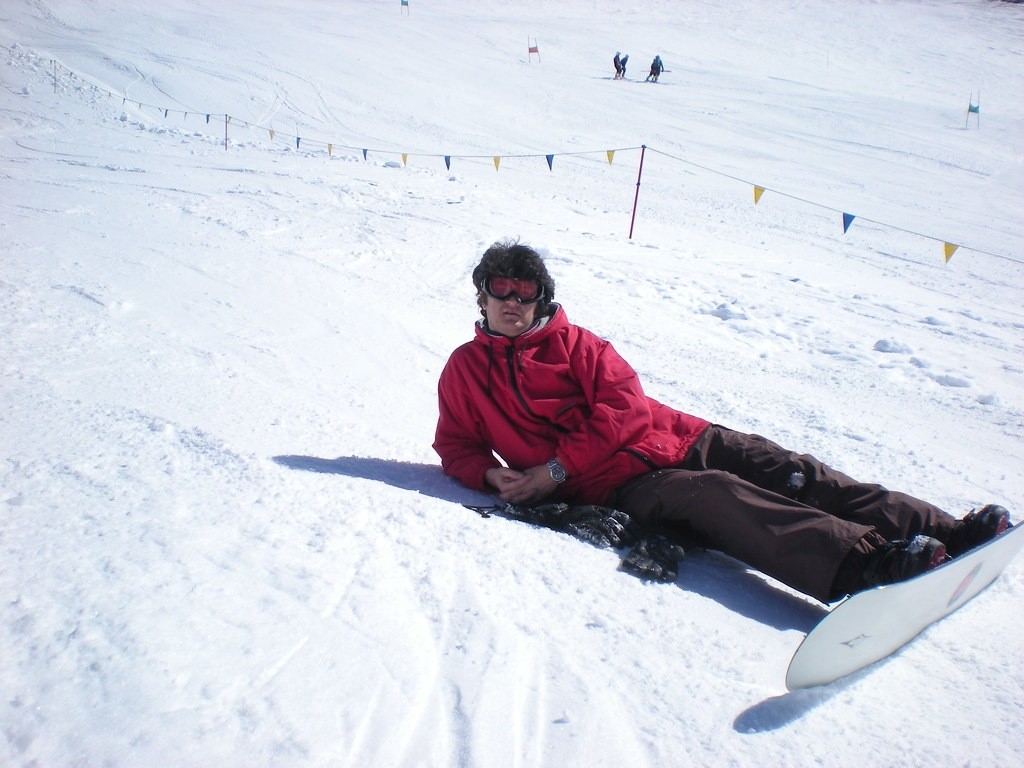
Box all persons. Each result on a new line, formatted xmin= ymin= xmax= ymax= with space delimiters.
xmin=614 ymin=51 xmax=622 ymax=79
xmin=646 ymin=55 xmax=664 ymax=82
xmin=432 ymin=244 xmax=1009 ymax=605
xmin=621 ymin=55 xmax=629 ymax=77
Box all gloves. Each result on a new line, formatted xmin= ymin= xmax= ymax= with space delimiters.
xmin=623 ymin=530 xmax=685 ymax=582
xmin=544 ymin=497 xmax=644 ymax=551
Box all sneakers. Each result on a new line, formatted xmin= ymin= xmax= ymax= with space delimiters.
xmin=862 ymin=535 xmax=946 ymax=584
xmin=948 ymin=504 xmax=1010 ymax=556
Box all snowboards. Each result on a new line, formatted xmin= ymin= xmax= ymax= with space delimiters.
xmin=784 ymin=520 xmax=1023 ymax=692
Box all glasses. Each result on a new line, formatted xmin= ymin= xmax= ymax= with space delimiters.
xmin=481 ymin=275 xmax=545 ymax=303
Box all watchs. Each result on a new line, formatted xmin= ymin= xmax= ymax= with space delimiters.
xmin=547 ymin=460 xmax=566 ymax=483
